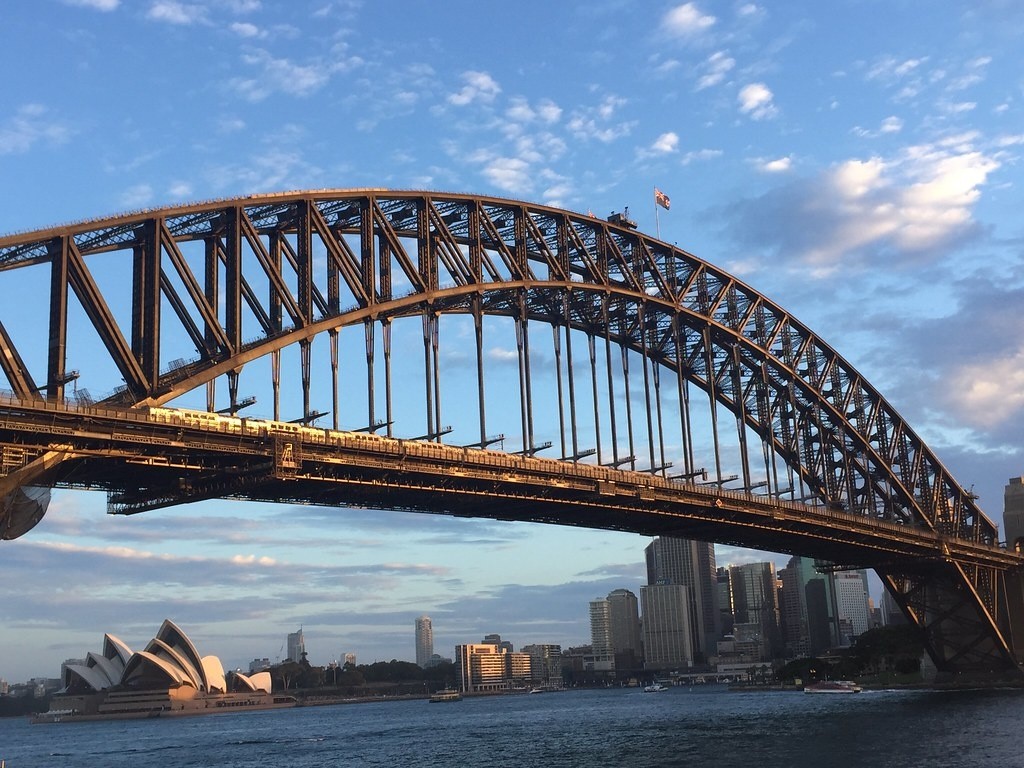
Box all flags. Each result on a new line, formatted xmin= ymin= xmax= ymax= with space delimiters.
xmin=654 ymin=187 xmax=670 ymax=211
xmin=589 ymin=211 xmax=596 ymax=219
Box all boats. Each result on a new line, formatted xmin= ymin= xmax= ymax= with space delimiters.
xmin=529 ymin=687 xmax=545 ymax=694
xmin=428 ymin=687 xmax=464 ymax=704
xmin=644 ymin=684 xmax=668 ymax=692
xmin=801 ymin=682 xmax=862 ymax=694
